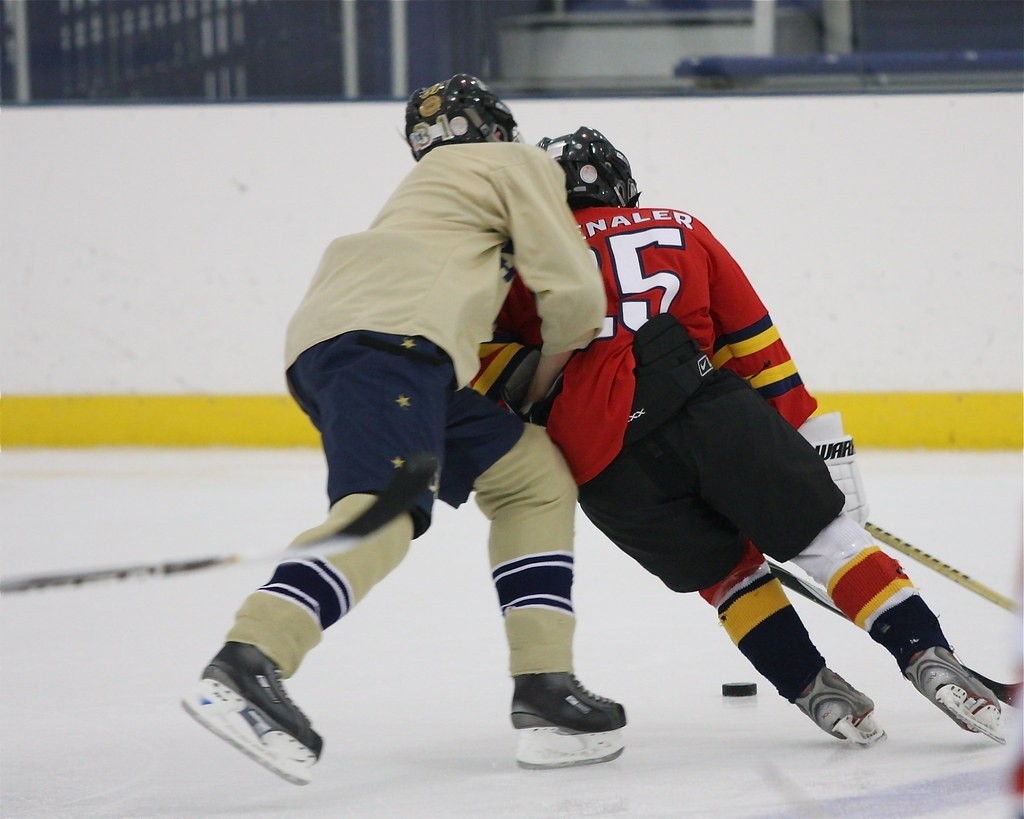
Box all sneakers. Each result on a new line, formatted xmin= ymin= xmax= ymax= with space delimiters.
xmin=181 ymin=641 xmax=323 ymax=785
xmin=794 ymin=666 xmax=888 ymax=749
xmin=512 ymin=671 xmax=627 ymax=768
xmin=903 ymin=645 xmax=1007 ymax=745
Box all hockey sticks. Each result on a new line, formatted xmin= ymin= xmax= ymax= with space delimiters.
xmin=2 ymin=453 xmax=439 ymax=598
xmin=863 ymin=521 xmax=1024 ymax=619
xmin=765 ymin=557 xmax=1024 ymax=710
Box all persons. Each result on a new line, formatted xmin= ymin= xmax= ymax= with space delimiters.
xmin=180 ymin=71 xmax=630 ymax=788
xmin=464 ymin=124 xmax=1011 ymax=751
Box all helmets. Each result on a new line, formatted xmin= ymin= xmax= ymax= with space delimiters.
xmin=534 ymin=126 xmax=643 ymax=208
xmin=405 ymin=73 xmax=518 ymax=162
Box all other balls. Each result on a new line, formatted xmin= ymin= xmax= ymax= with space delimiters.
xmin=721 ymin=681 xmax=758 ymax=697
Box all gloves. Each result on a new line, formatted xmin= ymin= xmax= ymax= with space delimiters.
xmin=795 ymin=411 xmax=866 ymax=529
xmin=499 ymin=347 xmax=564 ymax=427
